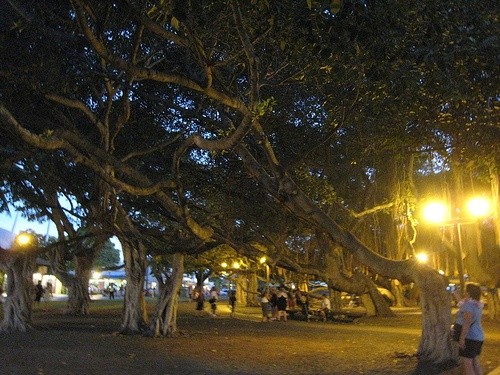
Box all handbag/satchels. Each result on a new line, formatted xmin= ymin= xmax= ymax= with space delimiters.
xmin=453 ymin=323 xmax=467 ymax=342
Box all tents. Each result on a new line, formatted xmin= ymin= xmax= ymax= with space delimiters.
xmin=100 ymin=253 xmax=210 ymax=297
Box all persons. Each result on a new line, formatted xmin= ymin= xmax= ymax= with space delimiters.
xmin=0 ymin=280 xmax=500 ymax=328
xmin=454 ymin=283 xmax=485 ymax=375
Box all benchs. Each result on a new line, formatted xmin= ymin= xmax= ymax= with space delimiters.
xmin=288 ymin=308 xmax=362 ymax=323
